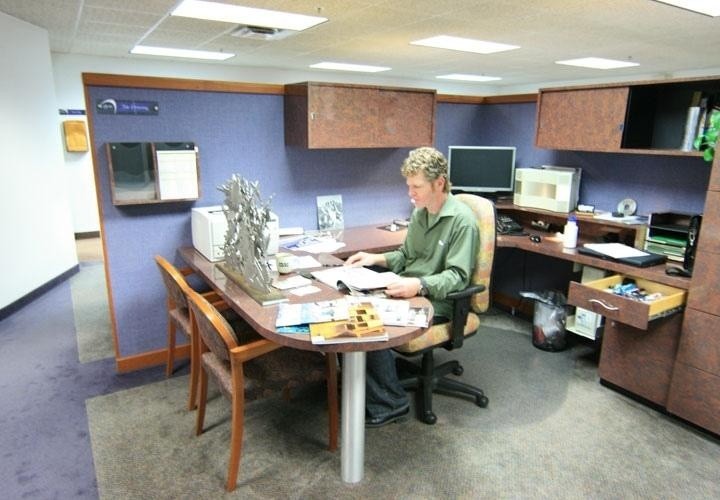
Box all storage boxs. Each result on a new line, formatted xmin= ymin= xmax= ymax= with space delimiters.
xmin=190 ymin=203 xmax=278 ymax=262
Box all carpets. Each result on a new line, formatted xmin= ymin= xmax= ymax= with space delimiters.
xmin=70 ymin=263 xmax=115 ymax=365
xmin=84 ymin=327 xmax=720 ymax=499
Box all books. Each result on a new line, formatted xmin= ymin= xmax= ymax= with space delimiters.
xmin=309 ymin=303 xmax=390 ymax=345
xmin=316 ymin=194 xmax=343 ymax=230
xmin=644 ymin=233 xmax=688 ymax=264
xmin=310 ymin=265 xmax=400 ymax=296
xmin=156 ymin=150 xmax=198 ymax=199
xmin=681 ymin=90 xmax=720 ymax=152
xmin=593 ymin=211 xmax=649 ymax=226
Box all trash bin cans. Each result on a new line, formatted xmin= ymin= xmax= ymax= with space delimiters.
xmin=532 ymin=289 xmax=574 ymax=353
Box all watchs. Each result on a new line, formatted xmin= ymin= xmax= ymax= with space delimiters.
xmin=419 ymin=279 xmax=427 ymax=298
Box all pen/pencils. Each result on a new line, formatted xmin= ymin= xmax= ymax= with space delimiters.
xmin=323 ymin=264 xmax=344 ymax=267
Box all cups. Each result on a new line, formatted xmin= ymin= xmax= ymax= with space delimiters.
xmin=275 ymin=252 xmax=295 ymax=275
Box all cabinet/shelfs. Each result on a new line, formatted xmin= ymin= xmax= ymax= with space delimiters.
xmin=489 ymin=198 xmax=689 ymax=291
xmin=105 ymin=142 xmax=202 ymax=206
xmin=535 ymin=75 xmax=720 ymax=157
xmin=565 ymin=274 xmax=688 ymax=416
xmin=665 ymin=136 xmax=720 ymax=438
xmin=284 ymin=82 xmax=436 ymax=151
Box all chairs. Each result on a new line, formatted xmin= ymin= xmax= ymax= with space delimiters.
xmin=182 ymin=287 xmax=338 ymax=492
xmin=152 ymin=255 xmax=267 ymax=411
xmin=391 ymin=193 xmax=496 ymax=425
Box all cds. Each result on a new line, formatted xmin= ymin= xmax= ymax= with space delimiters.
xmin=617 ymin=198 xmax=637 ymax=216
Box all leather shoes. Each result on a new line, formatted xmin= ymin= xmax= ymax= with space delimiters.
xmin=364 ymin=404 xmax=411 ymax=428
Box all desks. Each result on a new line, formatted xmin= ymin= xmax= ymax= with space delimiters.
xmin=175 ymin=246 xmax=435 ymax=484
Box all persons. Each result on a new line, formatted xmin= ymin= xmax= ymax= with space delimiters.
xmin=322 ymin=146 xmax=479 ymax=430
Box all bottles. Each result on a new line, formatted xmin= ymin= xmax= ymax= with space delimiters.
xmin=563 ymin=214 xmax=578 ymax=250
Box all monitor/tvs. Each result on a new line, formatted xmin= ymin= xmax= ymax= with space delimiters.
xmin=447 ymin=145 xmax=516 ymax=205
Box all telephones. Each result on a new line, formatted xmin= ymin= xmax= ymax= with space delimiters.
xmin=496 ymin=214 xmax=523 ymax=234
